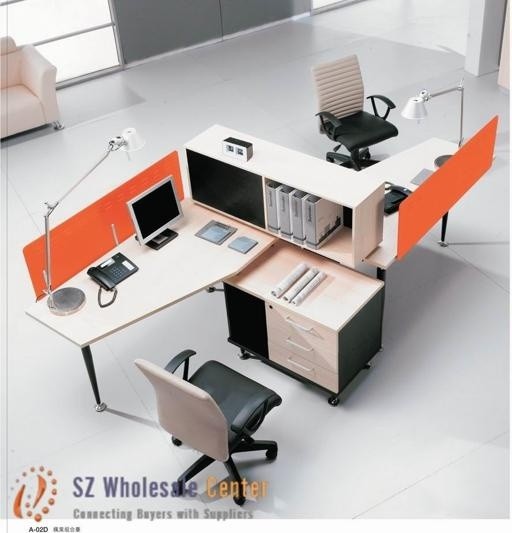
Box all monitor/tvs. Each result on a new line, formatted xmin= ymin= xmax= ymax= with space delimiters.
xmin=126 ymin=175 xmax=185 ymax=251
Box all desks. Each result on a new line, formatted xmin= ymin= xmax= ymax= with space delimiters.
xmin=25 ymin=200 xmax=280 ymax=412
xmin=349 ymin=111 xmax=498 ymax=264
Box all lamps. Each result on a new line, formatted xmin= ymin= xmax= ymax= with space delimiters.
xmin=41 ymin=127 xmax=147 ymax=317
xmin=401 ymin=83 xmax=465 ymax=168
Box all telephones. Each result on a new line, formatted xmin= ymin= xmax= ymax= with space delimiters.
xmin=87 ymin=252 xmax=139 ymax=307
xmin=383 ymin=180 xmax=414 ymax=218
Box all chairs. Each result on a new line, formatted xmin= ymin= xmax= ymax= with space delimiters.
xmin=1 ymin=34 xmax=66 ymax=140
xmin=135 ymin=348 xmax=283 ymax=506
xmin=311 ymin=52 xmax=399 ymax=172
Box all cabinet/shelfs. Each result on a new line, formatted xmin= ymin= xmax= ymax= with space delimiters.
xmin=223 ymin=242 xmax=387 ymax=409
xmin=184 ymin=124 xmax=385 ymax=271
